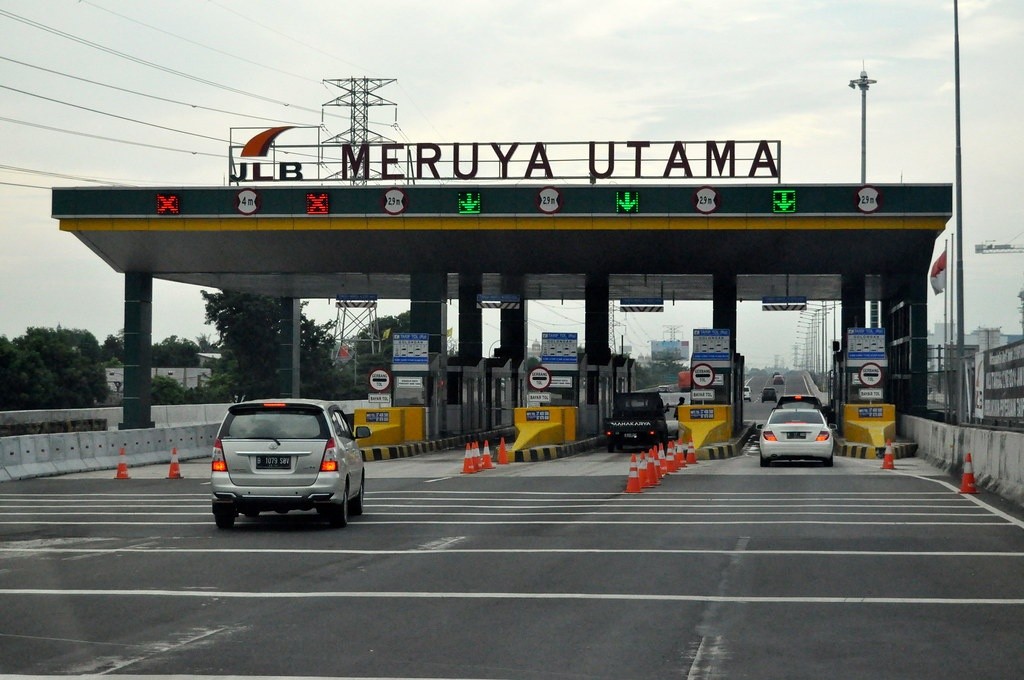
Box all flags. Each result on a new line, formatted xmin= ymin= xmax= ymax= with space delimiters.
xmin=930 ymin=251 xmax=946 ymax=295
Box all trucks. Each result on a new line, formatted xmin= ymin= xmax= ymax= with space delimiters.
xmin=678 ymin=370 xmax=692 ymax=393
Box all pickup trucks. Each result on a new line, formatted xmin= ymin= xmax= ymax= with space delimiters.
xmin=602 ymin=391 xmax=669 ymax=454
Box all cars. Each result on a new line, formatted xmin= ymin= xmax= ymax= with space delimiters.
xmin=760 ymin=387 xmax=779 ymax=403
xmin=210 ymin=398 xmax=372 ymax=527
xmin=743 ymin=386 xmax=752 ymax=401
xmin=772 ymin=371 xmax=785 ymax=385
xmin=755 ymin=394 xmax=838 ymax=468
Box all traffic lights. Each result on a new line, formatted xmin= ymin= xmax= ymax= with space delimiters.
xmin=615 ymin=190 xmax=640 ymax=213
xmin=307 ymin=193 xmax=329 ymax=215
xmin=773 ymin=190 xmax=797 ymax=213
xmin=156 ymin=193 xmax=179 ymax=215
xmin=458 ymin=191 xmax=481 ymax=214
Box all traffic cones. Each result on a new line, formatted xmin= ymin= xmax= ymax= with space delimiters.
xmin=957 ymin=453 xmax=981 ymax=494
xmin=114 ymin=447 xmax=132 ymax=479
xmin=498 ymin=437 xmax=510 ymax=464
xmin=165 ymin=447 xmax=183 ymax=478
xmin=625 ymin=435 xmax=698 ymax=493
xmin=461 ymin=440 xmax=497 ymax=474
xmin=879 ymin=438 xmax=896 ymax=470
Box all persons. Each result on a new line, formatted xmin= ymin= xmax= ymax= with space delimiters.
xmin=673 ymin=397 xmax=685 ymax=420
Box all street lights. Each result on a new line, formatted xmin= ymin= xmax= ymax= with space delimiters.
xmin=849 ymin=60 xmax=877 ymax=184
